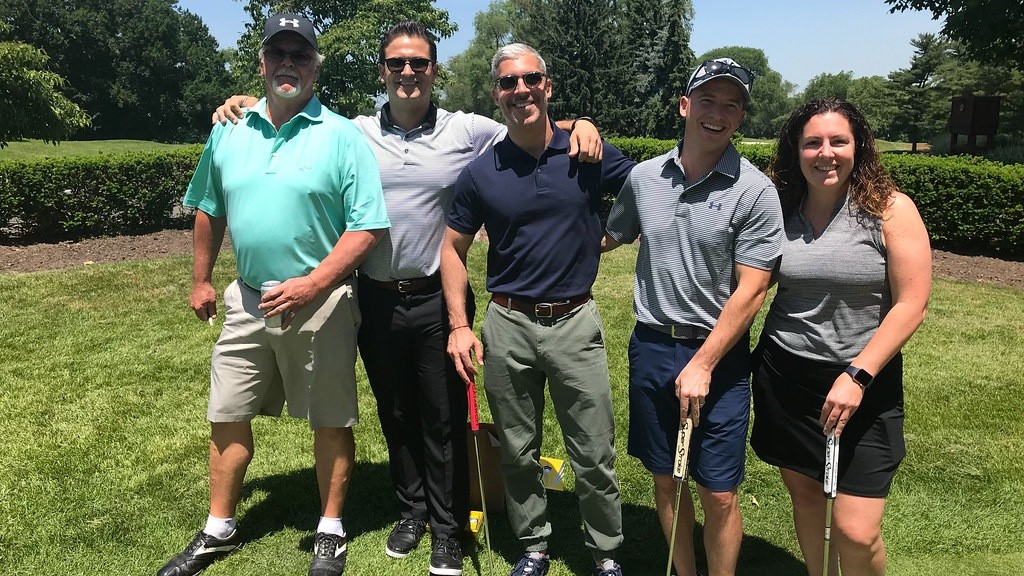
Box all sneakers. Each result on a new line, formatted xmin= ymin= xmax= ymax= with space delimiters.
xmin=308 ymin=527 xmax=347 ymax=576
xmin=385 ymin=519 xmax=428 ymax=558
xmin=429 ymin=536 xmax=463 ymax=576
xmin=592 ymin=550 xmax=622 ymax=576
xmin=155 ymin=527 xmax=242 ymax=576
xmin=509 ymin=553 xmax=549 ymax=576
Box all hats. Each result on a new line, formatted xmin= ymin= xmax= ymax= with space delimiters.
xmin=686 ymin=58 xmax=752 ymax=104
xmin=261 ymin=14 xmax=318 ymax=51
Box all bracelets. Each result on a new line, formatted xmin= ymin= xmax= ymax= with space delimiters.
xmin=449 ymin=324 xmax=469 ymax=335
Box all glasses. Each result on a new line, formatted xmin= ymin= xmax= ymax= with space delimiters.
xmin=261 ymin=48 xmax=317 ymax=66
xmin=382 ymin=57 xmax=437 ymax=73
xmin=685 ymin=60 xmax=755 ymax=98
xmin=494 ymin=71 xmax=548 ymax=90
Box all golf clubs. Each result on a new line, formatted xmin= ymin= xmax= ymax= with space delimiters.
xmin=460 ymin=347 xmax=497 ymax=576
xmin=822 ymin=421 xmax=840 ymax=576
xmin=665 ymin=400 xmax=695 ymax=576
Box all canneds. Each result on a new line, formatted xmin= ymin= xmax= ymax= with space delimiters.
xmin=260 ymin=280 xmax=285 ymax=328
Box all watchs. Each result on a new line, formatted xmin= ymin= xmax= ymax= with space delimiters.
xmin=843 ymin=366 xmax=874 ymax=389
xmin=572 ymin=116 xmax=597 ymax=131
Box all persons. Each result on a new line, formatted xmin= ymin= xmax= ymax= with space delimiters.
xmin=438 ymin=42 xmax=643 ymax=576
xmin=597 ymin=54 xmax=789 ymax=576
xmin=156 ymin=10 xmax=391 ymax=576
xmin=747 ymin=100 xmax=932 ymax=576
xmin=211 ymin=21 xmax=604 ymax=575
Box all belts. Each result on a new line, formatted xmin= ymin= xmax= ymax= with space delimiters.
xmin=358 ymin=272 xmax=443 ymax=292
xmin=492 ymin=289 xmax=592 ymax=318
xmin=646 ymin=323 xmax=710 ymax=339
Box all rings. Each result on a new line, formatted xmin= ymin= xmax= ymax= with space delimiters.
xmin=290 ymin=311 xmax=295 ymax=318
xmin=699 ymin=402 xmax=705 ymax=406
